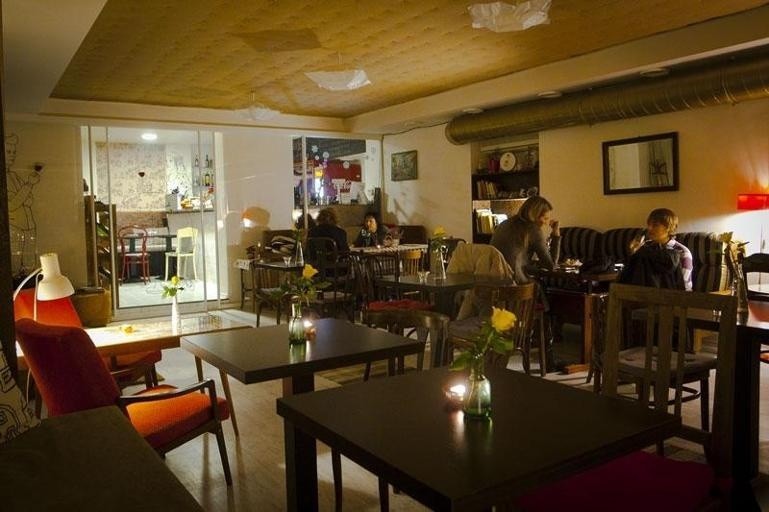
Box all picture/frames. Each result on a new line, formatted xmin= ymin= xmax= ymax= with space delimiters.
xmin=390 ymin=149 xmax=419 ymax=181
xmin=600 ymin=130 xmax=680 ymax=196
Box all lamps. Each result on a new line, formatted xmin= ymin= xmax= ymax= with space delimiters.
xmin=232 ymin=92 xmax=281 ymax=124
xmin=304 ymin=66 xmax=373 ymax=92
xmin=13 ymin=252 xmax=76 ymax=305
xmin=468 ymin=0 xmax=552 ymax=33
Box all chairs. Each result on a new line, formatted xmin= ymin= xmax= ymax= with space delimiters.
xmin=117 ymin=224 xmax=159 ymax=284
xmin=330 ymin=309 xmax=451 ymax=512
xmin=237 ymin=223 xmax=744 ymax=461
xmin=740 ymin=251 xmax=768 ymax=366
xmin=14 ymin=316 xmax=235 ymax=490
xmin=12 ymin=287 xmax=162 ymax=419
xmin=164 ymin=227 xmax=200 ymax=282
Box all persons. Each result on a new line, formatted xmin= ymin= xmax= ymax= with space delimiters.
xmin=296 ymin=214 xmax=315 ymax=230
xmin=618 ymin=208 xmax=693 ymax=291
xmin=488 ymin=196 xmax=561 ymax=283
xmin=307 ymin=207 xmax=350 ymax=262
xmin=355 ymin=211 xmax=391 ymax=246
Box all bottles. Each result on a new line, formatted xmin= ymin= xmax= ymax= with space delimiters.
xmin=193 ymin=153 xmax=214 ymax=186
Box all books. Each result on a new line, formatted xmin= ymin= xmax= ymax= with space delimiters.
xmin=476 ymin=178 xmax=497 ymax=200
xmin=475 ymin=208 xmax=508 ymax=233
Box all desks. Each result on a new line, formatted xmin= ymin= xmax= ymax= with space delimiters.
xmin=180 ymin=316 xmax=426 ymax=512
xmin=14 ymin=310 xmax=255 ymax=438
xmin=274 ymin=361 xmax=683 ymax=512
xmin=633 ymin=293 xmax=768 ymax=479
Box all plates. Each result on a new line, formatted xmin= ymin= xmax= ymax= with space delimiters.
xmin=499 ymin=152 xmax=515 ymax=171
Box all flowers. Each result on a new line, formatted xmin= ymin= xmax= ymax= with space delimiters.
xmin=162 ymin=276 xmax=186 ymax=297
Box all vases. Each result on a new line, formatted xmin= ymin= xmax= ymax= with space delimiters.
xmin=171 ymin=295 xmax=183 ymax=325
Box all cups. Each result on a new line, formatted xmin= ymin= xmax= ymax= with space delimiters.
xmin=488 ymin=159 xmax=498 ymax=171
xmin=282 ymin=256 xmax=292 ymax=264
xmin=417 ymin=271 xmax=430 ymax=280
